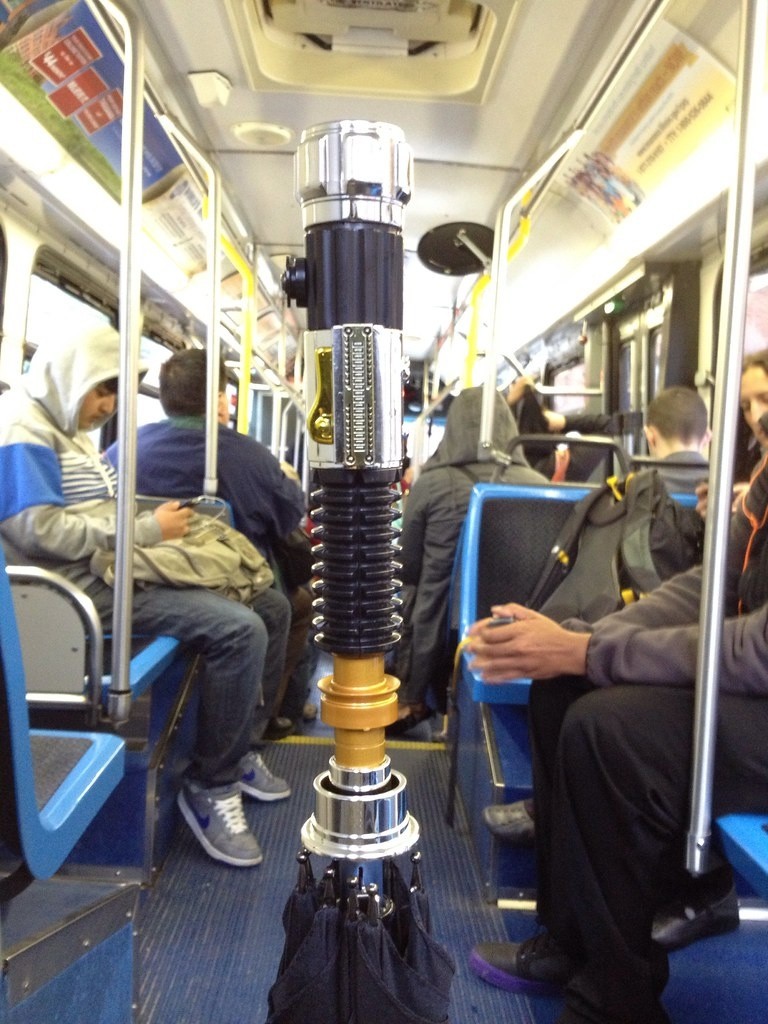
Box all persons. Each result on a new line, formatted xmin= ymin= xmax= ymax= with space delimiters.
xmin=694 ymin=349 xmax=767 ymax=521
xmin=635 ymin=387 xmax=708 ymax=497
xmin=299 ymin=456 xmax=415 ymax=550
xmin=0 ymin=321 xmax=292 ymax=868
xmin=396 ymin=386 xmax=551 ymax=705
xmin=506 ymin=378 xmax=615 ymax=483
xmin=98 ymin=348 xmax=307 ymax=741
xmin=465 ymin=409 xmax=768 ymax=1023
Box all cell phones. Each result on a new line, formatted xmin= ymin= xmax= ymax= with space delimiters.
xmin=177 ymin=499 xmax=201 ymax=510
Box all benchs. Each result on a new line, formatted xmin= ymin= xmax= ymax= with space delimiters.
xmin=458 ymin=483 xmax=699 ymax=702
xmin=9 ymin=497 xmax=228 ymax=719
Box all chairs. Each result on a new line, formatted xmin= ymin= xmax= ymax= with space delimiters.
xmin=0 ymin=554 xmax=128 ymax=883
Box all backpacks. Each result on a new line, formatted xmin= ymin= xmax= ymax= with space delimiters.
xmin=524 ymin=470 xmax=703 ymax=626
xmin=106 ymin=499 xmax=274 ymax=603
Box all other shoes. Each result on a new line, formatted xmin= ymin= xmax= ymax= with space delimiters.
xmin=482 ymin=798 xmax=537 ymax=842
xmin=304 ymin=703 xmax=318 ymax=720
xmin=649 ymin=885 xmax=739 ymax=949
xmin=385 ymin=706 xmax=433 ymax=739
xmin=263 ymin=717 xmax=294 ymax=739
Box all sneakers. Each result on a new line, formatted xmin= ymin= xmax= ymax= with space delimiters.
xmin=468 ymin=934 xmax=579 ymax=995
xmin=237 ymin=753 xmax=291 ymax=801
xmin=177 ymin=781 xmax=264 ymax=866
